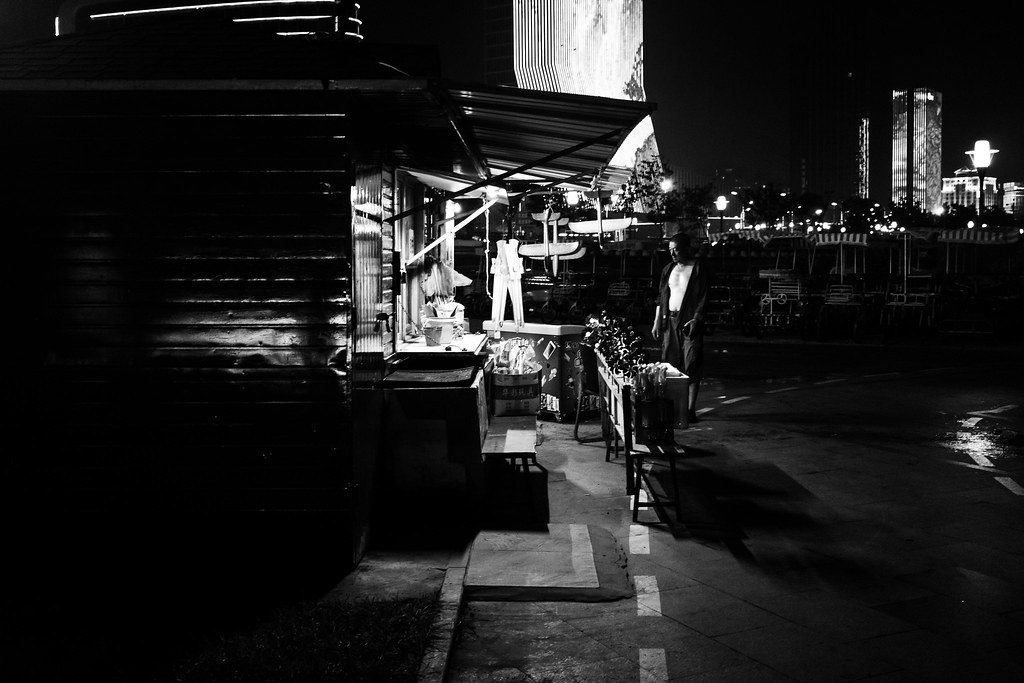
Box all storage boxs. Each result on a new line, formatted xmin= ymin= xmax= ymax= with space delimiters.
xmin=631 ymin=364 xmax=689 ymax=428
xmin=489 ymin=364 xmax=542 ymax=415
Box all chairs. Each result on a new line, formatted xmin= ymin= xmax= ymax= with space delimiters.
xmin=573 ymin=342 xmax=621 ymax=458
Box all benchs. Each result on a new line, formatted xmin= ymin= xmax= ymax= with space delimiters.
xmin=482 ymin=416 xmax=537 ymax=485
xmin=596 ymin=350 xmax=685 ymax=522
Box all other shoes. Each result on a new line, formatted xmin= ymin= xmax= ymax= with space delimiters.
xmin=688 ymin=409 xmax=697 ymax=423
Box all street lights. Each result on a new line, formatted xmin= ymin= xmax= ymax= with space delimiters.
xmin=713 ymin=194 xmax=730 ymax=234
xmin=966 ymin=141 xmax=1001 ymax=230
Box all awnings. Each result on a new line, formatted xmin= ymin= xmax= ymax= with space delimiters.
xmin=447 ymin=79 xmax=658 ymax=194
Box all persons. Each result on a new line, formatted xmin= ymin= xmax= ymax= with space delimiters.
xmin=651 ymin=233 xmax=711 ymax=424
